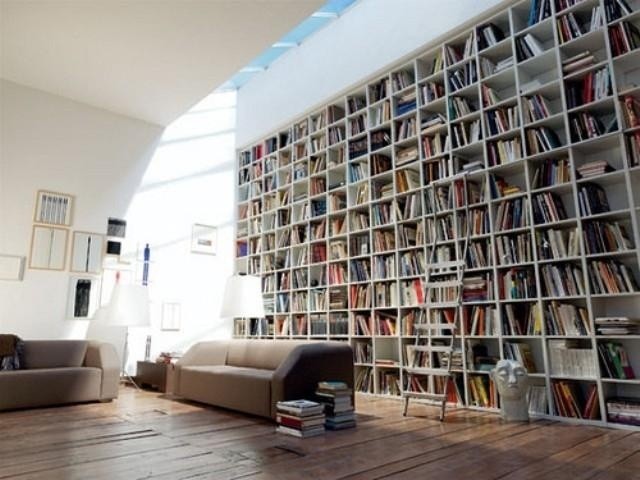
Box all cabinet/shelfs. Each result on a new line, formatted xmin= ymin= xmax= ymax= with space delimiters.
xmin=235 ymin=0 xmax=639 ymax=432
xmin=135 ymin=361 xmax=175 ymax=394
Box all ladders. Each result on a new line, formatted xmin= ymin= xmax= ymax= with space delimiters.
xmin=403 ymin=172 xmax=470 ymax=421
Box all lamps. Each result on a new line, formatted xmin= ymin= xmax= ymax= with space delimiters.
xmin=103 ymin=282 xmax=148 ymax=393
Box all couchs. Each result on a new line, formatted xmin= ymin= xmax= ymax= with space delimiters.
xmin=0 ymin=340 xmax=120 ymax=411
xmin=173 ymin=339 xmax=356 ymax=422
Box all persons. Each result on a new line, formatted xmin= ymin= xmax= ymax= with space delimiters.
xmin=489 ymin=360 xmax=529 ymax=421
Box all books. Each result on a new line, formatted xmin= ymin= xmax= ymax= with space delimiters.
xmin=275 ymin=381 xmax=357 ymax=438
xmin=233 ymin=0 xmax=640 ymax=426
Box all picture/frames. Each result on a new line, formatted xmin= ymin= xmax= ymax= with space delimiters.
xmin=28 ymin=191 xmax=106 ymax=275
